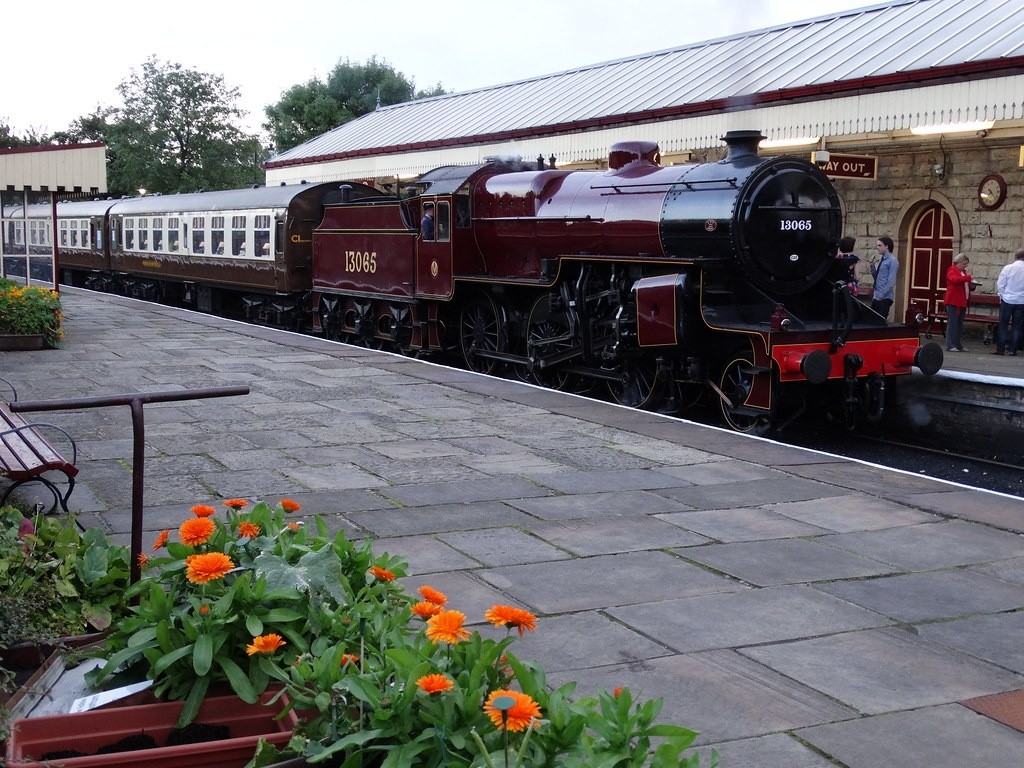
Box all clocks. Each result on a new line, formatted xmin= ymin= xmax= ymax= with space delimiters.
xmin=978 ymin=173 xmax=1007 ymax=211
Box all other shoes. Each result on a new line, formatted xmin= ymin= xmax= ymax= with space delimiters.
xmin=950 ymin=347 xmax=959 ymax=351
xmin=989 ymin=348 xmax=1004 ymax=355
xmin=1008 ymin=352 xmax=1017 ymax=356
xmin=961 ymin=347 xmax=969 ymax=351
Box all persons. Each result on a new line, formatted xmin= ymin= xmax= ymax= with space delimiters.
xmin=989 ymin=247 xmax=1024 ymax=355
xmin=831 ymin=236 xmax=862 ymax=299
xmin=869 ymin=236 xmax=898 ymax=318
xmin=945 ymin=253 xmax=978 ymax=352
xmin=421 ymin=202 xmax=438 ymax=241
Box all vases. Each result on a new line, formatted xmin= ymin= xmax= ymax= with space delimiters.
xmin=8 ymin=689 xmax=301 ymax=768
xmin=0 ymin=334 xmax=45 ymax=351
xmin=0 ymin=624 xmax=112 ymax=705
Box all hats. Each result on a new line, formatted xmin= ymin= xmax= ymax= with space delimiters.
xmin=423 ymin=203 xmax=434 ymax=211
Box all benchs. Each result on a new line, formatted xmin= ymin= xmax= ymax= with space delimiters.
xmin=0 ymin=378 xmax=85 ymax=532
xmin=925 ymin=291 xmax=1014 ymax=347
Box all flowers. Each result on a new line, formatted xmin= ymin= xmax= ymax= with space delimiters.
xmin=86 ymin=499 xmax=723 ymax=768
xmin=0 ymin=285 xmax=65 ymax=349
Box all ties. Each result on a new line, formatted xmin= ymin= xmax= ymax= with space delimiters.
xmin=873 ymin=257 xmax=888 ymax=288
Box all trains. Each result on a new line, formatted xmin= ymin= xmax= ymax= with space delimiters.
xmin=1 ymin=123 xmax=944 ymax=444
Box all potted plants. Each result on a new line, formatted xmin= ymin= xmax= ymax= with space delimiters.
xmin=0 ymin=506 xmax=131 ymax=700
